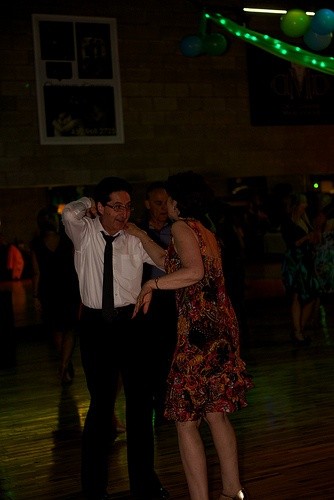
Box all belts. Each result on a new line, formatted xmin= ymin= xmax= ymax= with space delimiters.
xmin=83 ymin=304 xmax=135 ymax=318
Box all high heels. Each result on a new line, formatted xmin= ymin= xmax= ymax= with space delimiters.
xmin=290 ymin=333 xmax=312 ymax=346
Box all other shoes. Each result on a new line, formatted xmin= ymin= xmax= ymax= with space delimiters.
xmin=135 ymin=486 xmax=170 ymax=498
xmin=57 ymin=371 xmax=71 ymax=385
xmin=217 ymin=487 xmax=250 ymax=500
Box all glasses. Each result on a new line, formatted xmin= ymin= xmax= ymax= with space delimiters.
xmin=107 ymin=202 xmax=134 ymax=212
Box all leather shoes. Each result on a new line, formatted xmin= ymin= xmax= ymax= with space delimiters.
xmin=85 ymin=492 xmax=112 ymax=500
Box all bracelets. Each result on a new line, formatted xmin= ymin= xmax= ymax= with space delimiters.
xmin=155 ymin=278 xmax=159 ymax=289
xmin=32 ymin=294 xmax=37 ymax=298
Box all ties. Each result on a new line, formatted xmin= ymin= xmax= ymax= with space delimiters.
xmin=101 ymin=230 xmax=119 ymax=323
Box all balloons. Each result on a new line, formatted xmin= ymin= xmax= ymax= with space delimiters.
xmin=304 ymin=28 xmax=333 ymax=50
xmin=180 ymin=36 xmax=204 ymax=56
xmin=311 ymin=9 xmax=334 ymax=34
xmin=281 ymin=9 xmax=311 ymax=37
xmin=205 ymin=32 xmax=227 ymax=55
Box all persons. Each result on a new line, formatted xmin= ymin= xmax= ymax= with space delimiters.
xmin=31 ymin=206 xmax=81 ymax=383
xmin=63 ymin=177 xmax=173 ymax=500
xmin=139 ymin=180 xmax=178 ymax=422
xmin=245 ymin=180 xmax=334 ymax=343
xmin=126 ymin=171 xmax=249 ymax=500
